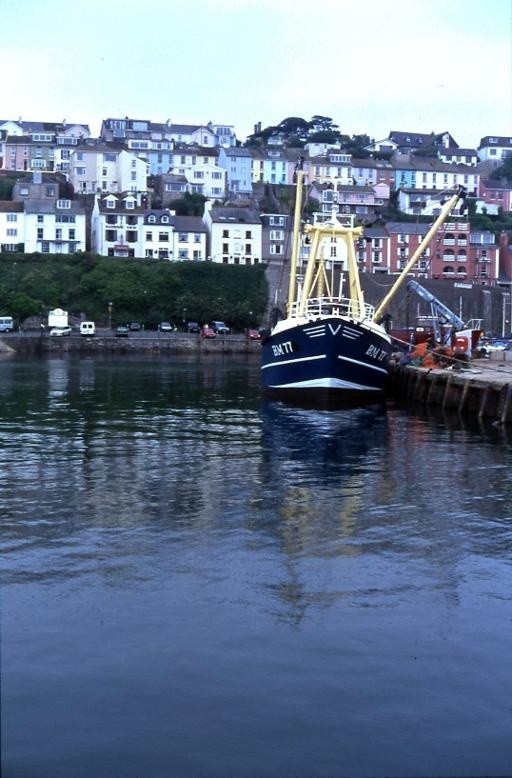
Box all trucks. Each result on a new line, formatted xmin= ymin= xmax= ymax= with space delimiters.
xmin=80 ymin=321 xmax=96 ymax=336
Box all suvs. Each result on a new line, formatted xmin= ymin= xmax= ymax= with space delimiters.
xmin=211 ymin=321 xmax=230 ymax=334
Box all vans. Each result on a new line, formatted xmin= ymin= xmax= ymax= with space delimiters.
xmin=0 ymin=316 xmax=14 ymax=333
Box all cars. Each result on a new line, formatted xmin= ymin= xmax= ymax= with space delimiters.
xmin=129 ymin=322 xmax=141 ymax=330
xmin=114 ymin=326 xmax=129 ymax=337
xmin=200 ymin=328 xmax=216 ymax=338
xmin=247 ymin=329 xmax=261 ymax=340
xmin=50 ymin=325 xmax=72 ymax=336
xmin=161 ymin=322 xmax=173 ymax=332
xmin=186 ymin=322 xmax=200 ymax=332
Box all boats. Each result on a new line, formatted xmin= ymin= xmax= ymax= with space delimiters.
xmin=388 ymin=279 xmax=485 ymax=356
xmin=253 ymin=402 xmax=390 ymax=572
xmin=259 ymin=156 xmax=466 ymax=411
